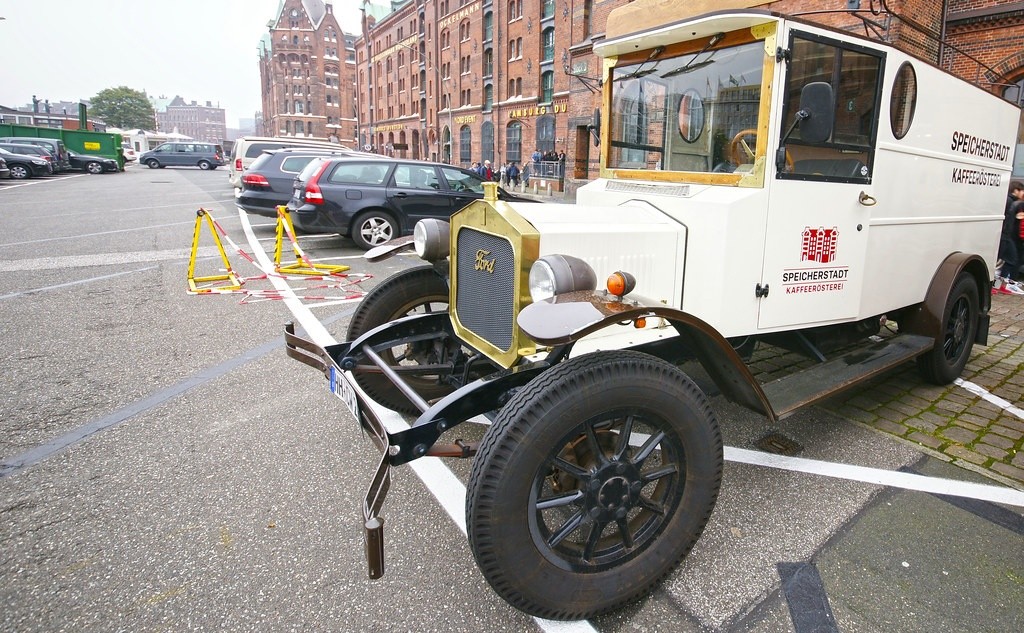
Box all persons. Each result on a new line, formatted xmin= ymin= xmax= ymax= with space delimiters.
xmin=992 ymin=181 xmax=1024 ymax=295
xmin=32 ymin=95 xmax=42 ymax=113
xmin=505 ymin=162 xmax=520 ymax=186
xmin=468 ymin=159 xmax=493 ymax=181
xmin=531 ymin=149 xmax=566 ymax=177
xmin=44 ymin=100 xmax=52 ymax=114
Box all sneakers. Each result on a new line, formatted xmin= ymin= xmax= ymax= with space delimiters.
xmin=1004 ymin=278 xmax=1022 ymax=287
xmin=1006 ymin=284 xmax=1024 ymax=294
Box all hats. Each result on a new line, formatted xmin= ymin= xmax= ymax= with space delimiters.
xmin=485 ymin=160 xmax=490 ymax=164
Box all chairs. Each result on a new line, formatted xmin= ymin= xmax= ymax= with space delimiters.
xmin=414 ymin=169 xmax=426 ymax=188
xmin=787 ymin=158 xmax=867 ymax=179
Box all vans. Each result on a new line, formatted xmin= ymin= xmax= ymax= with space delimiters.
xmin=227 ymin=135 xmax=356 ymax=188
xmin=140 ymin=141 xmax=229 ymax=171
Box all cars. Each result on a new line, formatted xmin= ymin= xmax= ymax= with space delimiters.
xmin=286 ymin=0 xmax=1022 ymax=622
xmin=0 ymin=136 xmax=72 ymax=181
xmin=64 ymin=147 xmax=120 ymax=174
xmin=121 ymin=142 xmax=138 ymax=163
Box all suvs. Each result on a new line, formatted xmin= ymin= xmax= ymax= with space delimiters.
xmin=233 ymin=148 xmax=385 ymax=236
xmin=282 ymin=156 xmax=545 ymax=252
